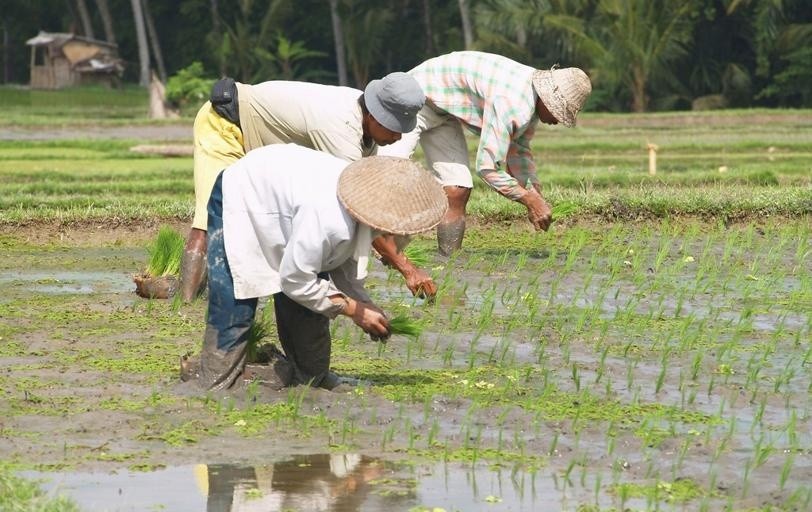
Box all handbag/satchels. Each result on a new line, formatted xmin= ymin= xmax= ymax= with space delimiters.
xmin=210 ymin=77 xmax=240 ymax=124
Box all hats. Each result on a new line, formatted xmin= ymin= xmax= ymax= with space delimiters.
xmin=532 ymin=62 xmax=592 ymax=128
xmin=337 ymin=156 xmax=449 ymax=235
xmin=364 ymin=71 xmax=426 ymax=133
